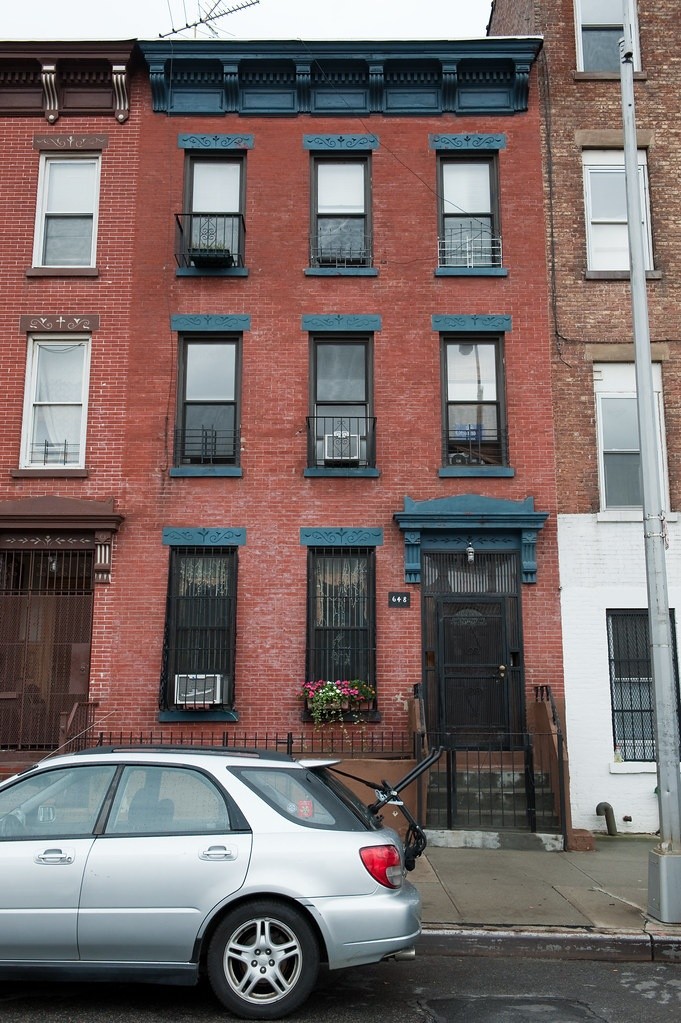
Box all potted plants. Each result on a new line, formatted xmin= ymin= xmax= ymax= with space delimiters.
xmin=188 ymin=240 xmax=233 ymax=268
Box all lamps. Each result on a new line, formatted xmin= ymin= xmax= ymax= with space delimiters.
xmin=466 ymin=546 xmax=475 ymax=564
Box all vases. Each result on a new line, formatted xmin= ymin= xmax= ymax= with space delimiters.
xmin=351 ymin=700 xmax=374 ymax=711
xmin=306 ymin=698 xmax=349 ymax=710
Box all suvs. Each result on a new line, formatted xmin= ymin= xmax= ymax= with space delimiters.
xmin=0 ymin=744 xmax=423 ymax=1022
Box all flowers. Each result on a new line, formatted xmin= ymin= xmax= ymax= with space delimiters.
xmin=348 ymin=679 xmax=376 ymax=743
xmin=301 ymin=679 xmax=352 ymax=758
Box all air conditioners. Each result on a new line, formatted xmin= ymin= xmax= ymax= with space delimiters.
xmin=174 ymin=674 xmax=229 ymax=705
xmin=449 ymin=453 xmax=485 ymax=464
xmin=324 ymin=434 xmax=360 ymax=461
